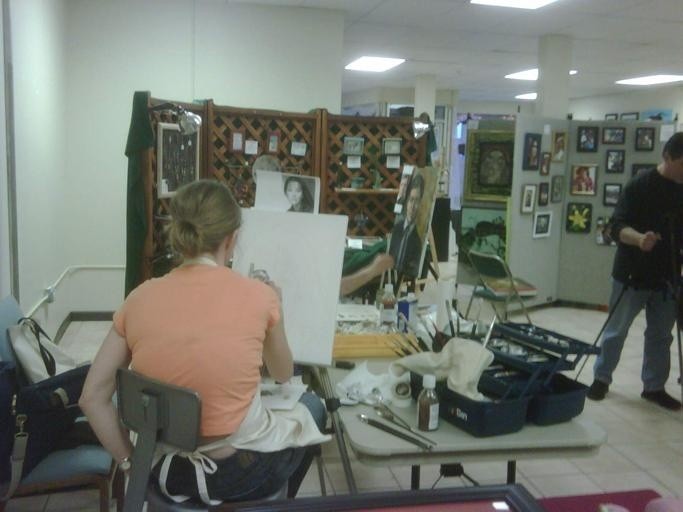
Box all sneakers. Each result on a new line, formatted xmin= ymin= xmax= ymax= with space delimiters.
xmin=637 ymin=388 xmax=682 ymax=411
xmin=587 ymin=378 xmax=610 ymax=401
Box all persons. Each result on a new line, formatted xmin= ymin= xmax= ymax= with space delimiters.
xmin=341 ymin=252 xmax=394 ymax=295
xmin=79 ymin=182 xmax=326 ymax=503
xmin=387 ymin=173 xmax=426 ymax=274
xmin=588 ymin=132 xmax=682 ymax=411
xmin=284 ymin=178 xmax=314 ymax=213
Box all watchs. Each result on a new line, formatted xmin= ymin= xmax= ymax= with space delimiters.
xmin=118 ymin=456 xmax=132 ymax=472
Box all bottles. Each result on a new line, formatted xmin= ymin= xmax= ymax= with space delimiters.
xmin=415 ymin=373 xmax=439 ymax=431
xmin=381 ymin=283 xmax=395 ymax=324
xmin=350 ymin=177 xmax=366 ymax=189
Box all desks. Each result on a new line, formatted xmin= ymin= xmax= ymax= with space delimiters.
xmin=330 ymin=349 xmax=606 ymax=490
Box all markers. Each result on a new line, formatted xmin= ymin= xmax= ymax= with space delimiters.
xmin=333 ymin=361 xmax=354 ymax=370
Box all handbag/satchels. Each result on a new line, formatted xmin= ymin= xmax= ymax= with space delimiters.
xmin=4 ymin=316 xmax=78 ymax=386
xmin=0 ymin=362 xmax=100 ymax=485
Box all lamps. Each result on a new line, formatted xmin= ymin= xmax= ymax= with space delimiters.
xmin=146 ymin=100 xmax=205 ymax=136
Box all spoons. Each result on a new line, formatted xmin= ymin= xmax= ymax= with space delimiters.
xmin=371 ymin=387 xmax=440 ymax=447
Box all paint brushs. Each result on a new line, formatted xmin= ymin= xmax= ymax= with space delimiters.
xmin=356 ymin=403 xmax=438 ymax=452
xmin=388 ymin=302 xmax=499 ymax=357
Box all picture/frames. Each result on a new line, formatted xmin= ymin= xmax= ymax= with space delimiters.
xmin=263 ymin=130 xmax=281 ymax=155
xmin=341 ymin=135 xmax=367 ymax=156
xmin=154 ymin=120 xmax=201 ymax=199
xmin=460 ymin=127 xmax=514 ymax=206
xmin=379 ymin=136 xmax=402 ymax=155
xmin=517 ymin=110 xmax=658 ymax=248
xmin=226 ymin=128 xmax=246 ymax=154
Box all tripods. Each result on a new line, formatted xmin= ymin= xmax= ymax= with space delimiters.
xmin=574 ymin=208 xmax=683 ymax=410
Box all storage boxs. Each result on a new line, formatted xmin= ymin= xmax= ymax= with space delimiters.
xmin=403 ymin=319 xmax=602 ymax=440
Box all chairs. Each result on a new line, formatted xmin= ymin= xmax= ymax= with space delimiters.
xmin=464 ymin=248 xmax=538 ymax=326
xmin=0 ymin=293 xmax=127 ymax=511
xmin=109 ymin=364 xmax=289 ymax=511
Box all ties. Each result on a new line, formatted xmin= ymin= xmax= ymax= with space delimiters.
xmin=395 ymin=225 xmax=410 ymax=278
